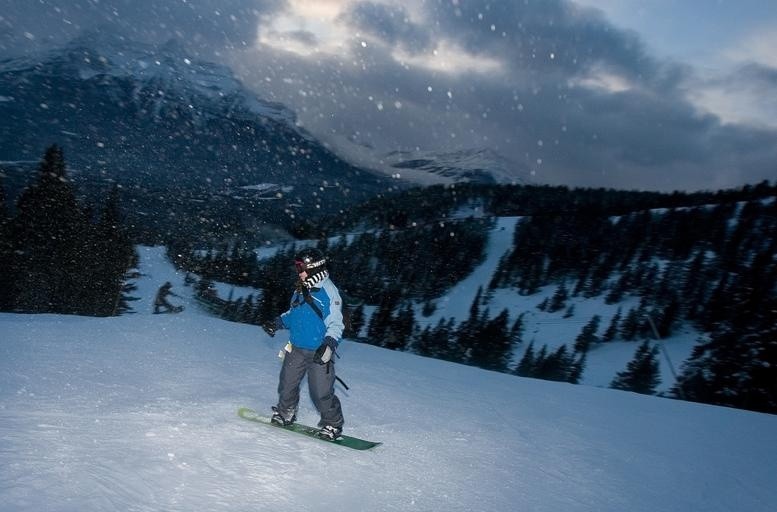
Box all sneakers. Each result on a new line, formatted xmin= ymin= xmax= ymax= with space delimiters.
xmin=319 ymin=425 xmax=342 ymax=440
xmin=271 ymin=407 xmax=295 ymax=426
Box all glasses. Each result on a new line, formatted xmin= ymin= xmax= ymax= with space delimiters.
xmin=295 ymin=263 xmax=306 ymax=273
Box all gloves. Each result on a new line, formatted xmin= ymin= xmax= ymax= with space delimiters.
xmin=313 ymin=344 xmax=333 ymax=365
xmin=262 ymin=321 xmax=276 ymax=336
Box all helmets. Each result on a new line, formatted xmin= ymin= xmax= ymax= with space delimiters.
xmin=295 ymin=247 xmax=327 ymax=274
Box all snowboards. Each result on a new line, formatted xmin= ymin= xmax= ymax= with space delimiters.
xmin=238 ymin=408 xmax=382 ymax=450
xmin=152 ymin=306 xmax=185 ymax=315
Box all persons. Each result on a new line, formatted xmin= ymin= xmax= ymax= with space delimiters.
xmin=153 ymin=279 xmax=176 ymax=313
xmin=261 ymin=245 xmax=345 ymax=441
